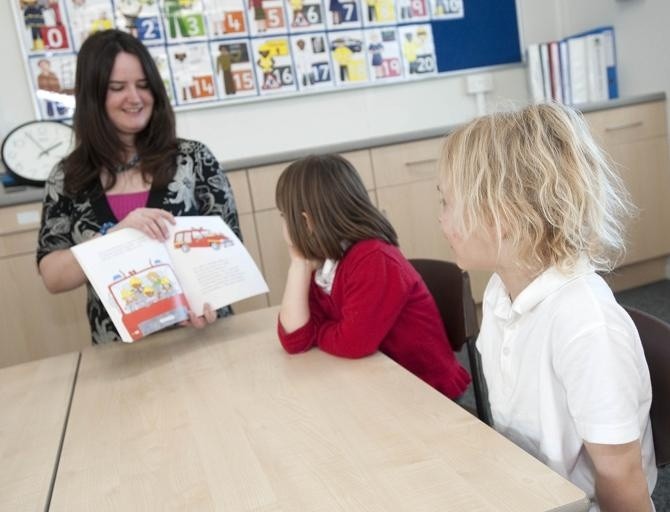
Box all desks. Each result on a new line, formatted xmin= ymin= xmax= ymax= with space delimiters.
xmin=0 ymin=303 xmax=590 ymax=512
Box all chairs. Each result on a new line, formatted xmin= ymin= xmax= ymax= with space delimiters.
xmin=625 ymin=303 xmax=669 ymax=469
xmin=408 ymin=259 xmax=491 ymax=427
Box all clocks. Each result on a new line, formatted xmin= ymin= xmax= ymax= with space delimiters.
xmin=0 ymin=118 xmax=79 ymax=187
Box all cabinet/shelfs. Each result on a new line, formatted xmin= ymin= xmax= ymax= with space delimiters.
xmin=1 ymin=93 xmax=670 ymax=368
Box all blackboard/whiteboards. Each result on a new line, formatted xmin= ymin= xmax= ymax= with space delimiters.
xmin=9 ymin=0 xmax=529 ymax=121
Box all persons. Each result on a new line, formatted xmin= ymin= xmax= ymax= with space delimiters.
xmin=436 ymin=103 xmax=658 ymax=512
xmin=37 ymin=29 xmax=242 ymax=348
xmin=275 ymin=152 xmax=471 ymax=405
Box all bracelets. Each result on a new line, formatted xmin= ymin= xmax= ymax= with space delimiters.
xmin=100 ymin=222 xmax=113 ymax=235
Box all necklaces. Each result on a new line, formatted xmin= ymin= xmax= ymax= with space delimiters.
xmin=111 ymin=154 xmax=139 ymax=172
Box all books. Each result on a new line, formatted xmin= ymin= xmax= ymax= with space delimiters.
xmin=71 ymin=215 xmax=270 ymax=344
